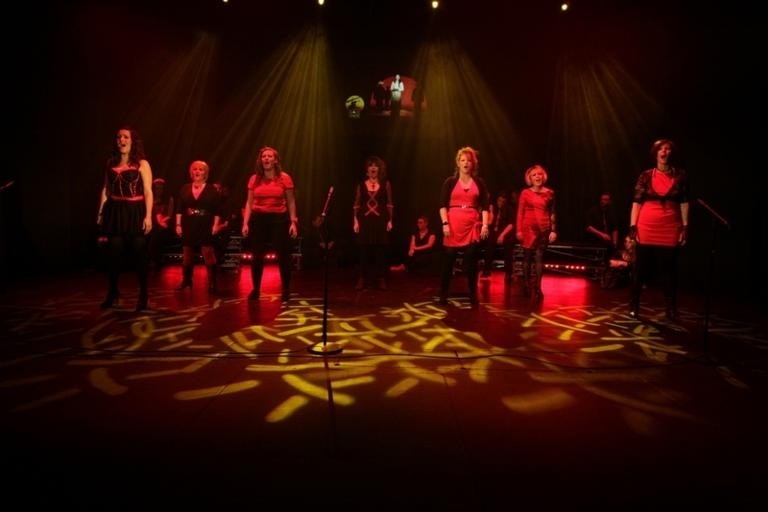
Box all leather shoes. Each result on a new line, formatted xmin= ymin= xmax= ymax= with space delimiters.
xmin=439 ymin=289 xmax=450 ymax=304
xmin=523 ymin=284 xmax=544 ymax=298
xmin=469 ymin=292 xmax=478 ymax=304
xmin=281 ymin=291 xmax=290 ymax=301
xmin=249 ymin=289 xmax=259 ymax=300
xmin=630 ymin=308 xmax=639 ymax=317
xmin=136 ymin=291 xmax=148 ymax=311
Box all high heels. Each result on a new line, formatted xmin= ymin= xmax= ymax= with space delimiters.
xmin=176 ymin=279 xmax=220 ymax=294
xmin=99 ymin=289 xmax=120 ymax=309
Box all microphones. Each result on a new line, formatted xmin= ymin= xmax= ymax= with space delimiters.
xmin=0 ymin=181 xmax=15 ymax=192
xmin=696 ymin=198 xmax=729 ymax=226
xmin=321 ymin=186 xmax=335 ymax=217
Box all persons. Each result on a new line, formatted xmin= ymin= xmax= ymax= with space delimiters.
xmin=93 ymin=125 xmax=154 ymax=311
xmin=350 ymin=152 xmax=392 ymax=292
xmin=390 ymin=215 xmax=437 ymax=276
xmin=239 ymin=145 xmax=300 ymax=304
xmin=373 ymin=81 xmax=386 ymax=114
xmin=304 ymin=215 xmax=338 ymax=268
xmin=148 ymin=179 xmax=232 ymax=273
xmin=582 ymin=191 xmax=624 ymax=278
xmin=480 ymin=191 xmax=514 ymax=287
xmin=436 ymin=146 xmax=484 ymax=304
xmin=390 ymin=75 xmax=405 ymax=118
xmin=515 ymin=164 xmax=556 ymax=301
xmin=626 ymin=137 xmax=695 ymax=324
xmin=173 ymin=160 xmax=223 ymax=295
